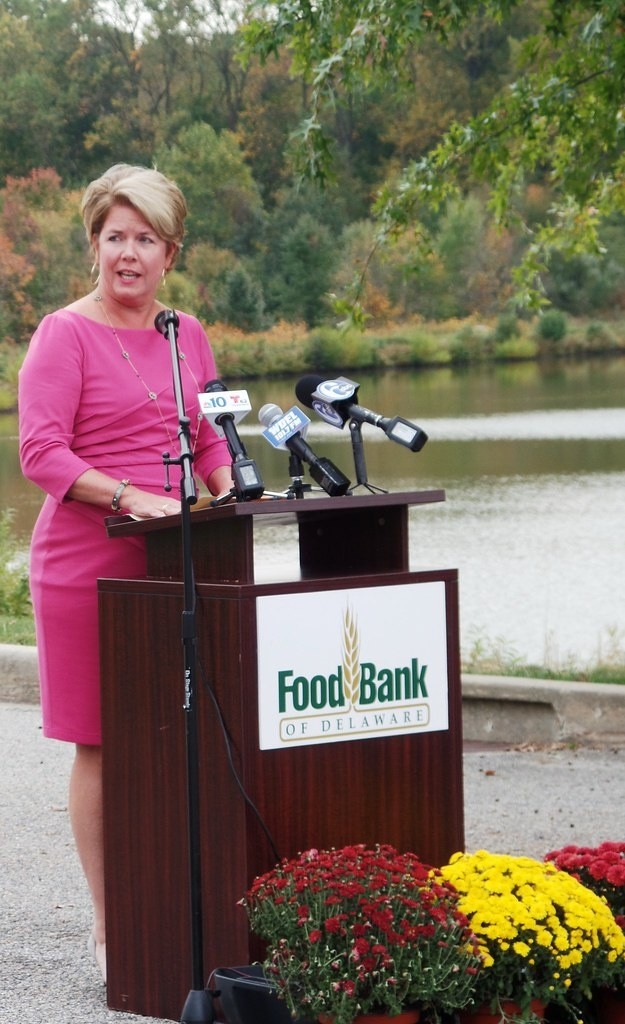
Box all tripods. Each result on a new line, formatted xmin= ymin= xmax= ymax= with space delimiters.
xmin=347 ymin=418 xmax=389 ymax=496
xmin=272 ymin=450 xmax=326 ymax=500
xmin=210 ymin=484 xmax=295 ymax=507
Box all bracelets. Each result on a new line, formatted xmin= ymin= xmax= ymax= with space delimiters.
xmin=112 ymin=478 xmax=130 ymax=512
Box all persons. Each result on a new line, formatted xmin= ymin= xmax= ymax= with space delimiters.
xmin=16 ymin=165 xmax=236 ymax=987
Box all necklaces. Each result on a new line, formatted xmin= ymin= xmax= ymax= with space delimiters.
xmin=95 ymin=296 xmax=204 ymax=455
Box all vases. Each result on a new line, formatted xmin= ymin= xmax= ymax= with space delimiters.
xmin=319 ymin=1011 xmax=418 ymax=1024
xmin=476 ymin=998 xmax=550 ymax=1024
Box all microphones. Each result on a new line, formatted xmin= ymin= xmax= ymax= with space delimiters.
xmin=198 ymin=379 xmax=265 ymax=500
xmin=155 ymin=311 xmax=180 ymax=340
xmin=295 ymin=375 xmax=429 ymax=452
xmin=258 ymin=404 xmax=351 ymax=498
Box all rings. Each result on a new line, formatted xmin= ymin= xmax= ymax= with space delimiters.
xmin=162 ymin=504 xmax=168 ymax=510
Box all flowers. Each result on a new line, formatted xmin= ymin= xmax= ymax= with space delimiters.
xmin=236 ymin=838 xmax=625 ymax=1024
